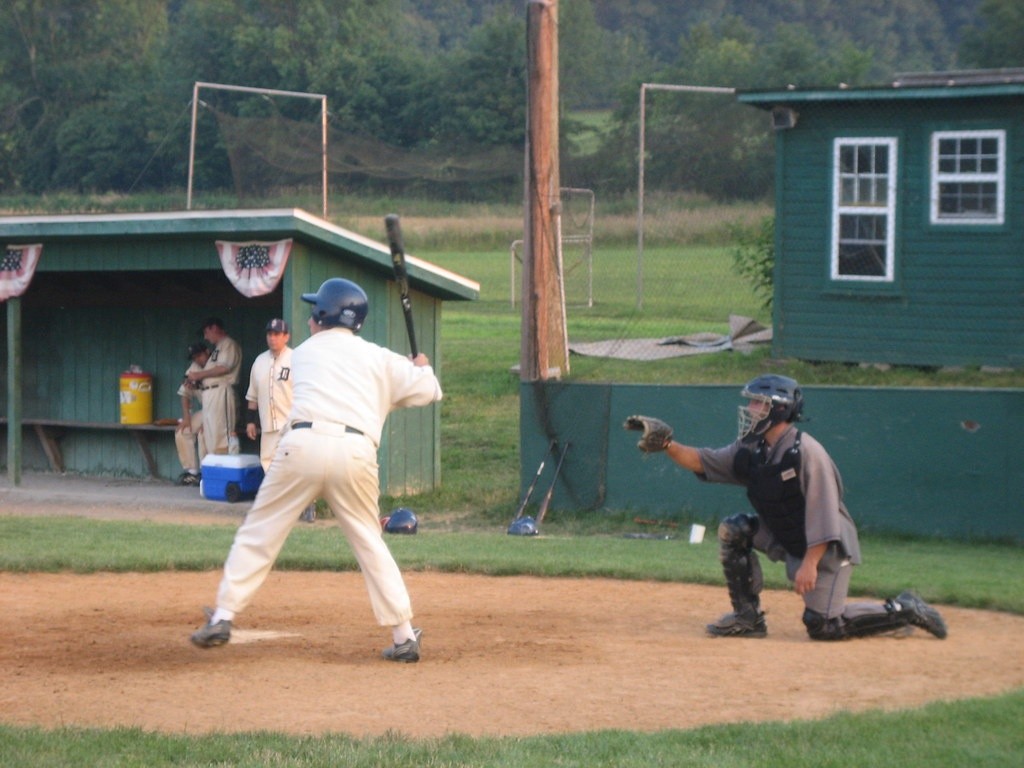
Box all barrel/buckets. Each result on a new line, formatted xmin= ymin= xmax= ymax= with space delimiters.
xmin=119 ymin=371 xmax=153 ymax=425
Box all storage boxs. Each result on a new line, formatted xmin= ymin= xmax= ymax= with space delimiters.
xmin=199 ymin=452 xmax=263 ymax=503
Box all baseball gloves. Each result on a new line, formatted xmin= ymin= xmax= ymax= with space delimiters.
xmin=622 ymin=414 xmax=675 ymax=452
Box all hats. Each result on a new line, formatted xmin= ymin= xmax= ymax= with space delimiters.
xmin=196 ymin=316 xmax=225 ymax=336
xmin=187 ymin=341 xmax=207 ymax=361
xmin=265 ymin=319 xmax=288 ymax=333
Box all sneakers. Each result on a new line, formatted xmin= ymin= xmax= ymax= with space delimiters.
xmin=189 ymin=606 xmax=233 ymax=647
xmin=381 ymin=627 xmax=422 ymax=663
xmin=175 ymin=471 xmax=201 ymax=487
xmin=705 ymin=607 xmax=768 ymax=638
xmin=894 ymin=589 xmax=947 ymax=640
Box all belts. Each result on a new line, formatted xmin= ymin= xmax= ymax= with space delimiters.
xmin=291 ymin=422 xmax=364 ymax=436
xmin=202 ymin=384 xmax=219 ymax=390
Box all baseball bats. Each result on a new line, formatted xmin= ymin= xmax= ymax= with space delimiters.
xmin=385 ymin=213 xmax=419 ymax=360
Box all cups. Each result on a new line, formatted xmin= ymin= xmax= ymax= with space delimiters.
xmin=689 ymin=524 xmax=706 ymax=544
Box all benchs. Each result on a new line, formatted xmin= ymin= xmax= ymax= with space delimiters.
xmin=0 ymin=418 xmax=262 ymax=479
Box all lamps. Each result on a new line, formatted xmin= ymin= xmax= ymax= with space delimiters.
xmin=769 ymin=106 xmax=801 ymax=130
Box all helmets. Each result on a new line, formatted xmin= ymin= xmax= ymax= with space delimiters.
xmin=737 ymin=374 xmax=811 ymax=445
xmin=381 ymin=506 xmax=418 ymax=534
xmin=507 ymin=515 xmax=539 ymax=537
xmin=299 ymin=278 xmax=370 ymax=333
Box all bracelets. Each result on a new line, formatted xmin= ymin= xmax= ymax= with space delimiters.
xmin=247 ymin=409 xmax=257 ymax=425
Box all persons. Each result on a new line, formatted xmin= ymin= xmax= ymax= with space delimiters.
xmin=245 ymin=318 xmax=294 ymax=477
xmin=663 ymin=374 xmax=948 ymax=640
xmin=187 ymin=278 xmax=443 ymax=664
xmin=174 ymin=317 xmax=241 ymax=487
